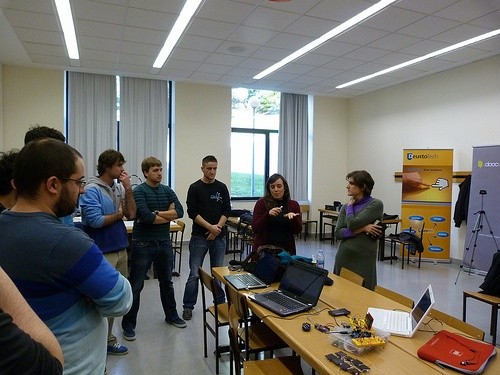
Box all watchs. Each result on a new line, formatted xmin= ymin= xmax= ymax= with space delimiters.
xmin=154 ymin=210 xmax=159 ymax=215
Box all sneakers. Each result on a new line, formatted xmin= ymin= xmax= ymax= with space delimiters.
xmin=172 ymin=318 xmax=187 ymax=327
xmin=107 ymin=343 xmax=128 ymax=355
xmin=183 ymin=308 xmax=192 ymax=319
xmin=123 ymin=328 xmax=136 ymax=341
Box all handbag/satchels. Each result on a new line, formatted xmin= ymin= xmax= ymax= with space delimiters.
xmin=417 ymin=330 xmax=496 ymax=375
xmin=241 ymin=245 xmax=290 ymax=284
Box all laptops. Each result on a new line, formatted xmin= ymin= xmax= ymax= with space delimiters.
xmin=366 ymin=283 xmax=435 ymax=338
xmin=247 ymin=259 xmax=330 ymax=319
xmin=221 ymin=249 xmax=280 ymax=290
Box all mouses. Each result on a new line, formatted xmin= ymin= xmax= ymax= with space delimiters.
xmin=303 ymin=323 xmax=310 ymax=331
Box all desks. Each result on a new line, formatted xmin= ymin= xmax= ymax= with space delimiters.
xmin=73 ymin=217 xmax=181 ymax=233
xmin=318 ymin=208 xmax=402 ymax=261
xmin=211 ymin=267 xmax=500 ymax=375
xmin=225 ymin=217 xmax=252 ymax=255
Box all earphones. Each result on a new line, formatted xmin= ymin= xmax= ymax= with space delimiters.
xmin=461 ymin=360 xmax=477 ymax=365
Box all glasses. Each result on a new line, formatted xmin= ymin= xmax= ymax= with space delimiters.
xmin=55 ymin=177 xmax=87 ymax=188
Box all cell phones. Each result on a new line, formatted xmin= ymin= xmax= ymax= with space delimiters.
xmin=329 ymin=308 xmax=350 ymax=317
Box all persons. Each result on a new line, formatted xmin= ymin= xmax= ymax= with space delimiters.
xmin=0 ymin=126 xmax=77 ymax=227
xmin=78 ymin=149 xmax=137 ymax=354
xmin=183 ymin=155 xmax=231 ymax=321
xmin=252 ymin=174 xmax=302 ymax=256
xmin=0 ymin=138 xmax=133 ymax=375
xmin=333 ymin=170 xmax=384 ymax=292
xmin=122 ymin=157 xmax=186 ymax=341
xmin=403 ymin=172 xmax=430 ymax=187
xmin=0 ymin=266 xmax=64 ymax=375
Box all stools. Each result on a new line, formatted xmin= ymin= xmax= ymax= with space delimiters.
xmin=323 ymin=222 xmax=336 ymax=245
xmin=463 ymin=291 xmax=500 ymax=346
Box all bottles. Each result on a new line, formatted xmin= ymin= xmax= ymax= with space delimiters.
xmin=316 ymin=250 xmax=324 ymax=268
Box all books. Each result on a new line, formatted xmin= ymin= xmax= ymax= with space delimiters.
xmin=366 ymin=220 xmax=388 ymax=240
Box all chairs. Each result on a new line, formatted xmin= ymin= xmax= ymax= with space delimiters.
xmin=225 ymin=283 xmax=290 ymax=375
xmin=227 ymin=304 xmax=293 ymax=375
xmin=297 ymin=205 xmax=317 ymax=242
xmin=340 ymin=267 xmax=365 ymax=287
xmin=390 ymin=221 xmax=425 ymax=269
xmin=172 ymin=220 xmax=185 ymax=273
xmin=374 ymin=285 xmax=414 ymax=309
xmin=428 ymin=307 xmax=486 ymax=340
xmin=197 ymin=266 xmax=241 ymax=358
